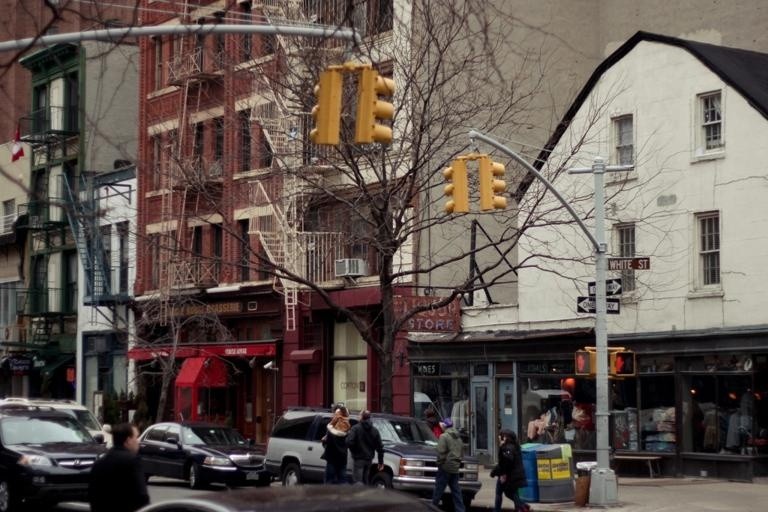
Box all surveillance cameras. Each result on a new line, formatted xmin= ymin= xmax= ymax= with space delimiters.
xmin=263 ymin=361 xmax=274 ymax=370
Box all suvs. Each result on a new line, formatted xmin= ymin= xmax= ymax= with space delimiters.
xmin=265 ymin=404 xmax=480 ymax=507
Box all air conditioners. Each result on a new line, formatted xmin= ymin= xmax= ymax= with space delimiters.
xmin=333 ymin=258 xmax=365 ymax=278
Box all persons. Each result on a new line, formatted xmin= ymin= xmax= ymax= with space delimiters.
xmin=87 ymin=422 xmax=149 ymax=512
xmin=701 ymin=393 xmax=754 ymax=457
xmin=344 ymin=409 xmax=384 ymax=486
xmin=497 ymin=431 xmax=531 ymax=512
xmin=321 ymin=404 xmax=349 ymax=448
xmin=319 ymin=416 xmax=351 ymax=485
xmin=432 ymin=418 xmax=466 ymax=512
xmin=424 ymin=409 xmax=443 ymax=439
xmin=490 ymin=429 xmax=528 ymax=511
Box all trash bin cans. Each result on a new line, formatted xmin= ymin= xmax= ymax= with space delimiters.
xmin=517 ymin=443 xmax=544 ymax=502
xmin=575 ymin=461 xmax=597 ymax=506
xmin=536 ymin=443 xmax=574 ymax=502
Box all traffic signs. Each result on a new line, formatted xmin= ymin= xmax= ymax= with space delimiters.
xmin=606 ymin=257 xmax=650 ymax=270
xmin=587 ymin=281 xmax=619 ymax=295
xmin=576 ymin=297 xmax=620 ymax=314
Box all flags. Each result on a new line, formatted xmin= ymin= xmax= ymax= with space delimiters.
xmin=12 ymin=121 xmax=24 ymax=162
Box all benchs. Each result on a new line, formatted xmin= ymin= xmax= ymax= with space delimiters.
xmin=610 ymin=455 xmax=662 ymax=479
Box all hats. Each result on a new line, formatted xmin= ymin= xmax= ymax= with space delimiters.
xmin=331 ymin=402 xmax=349 ymax=417
xmin=441 ymin=417 xmax=453 ymax=428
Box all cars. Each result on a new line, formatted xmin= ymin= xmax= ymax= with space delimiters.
xmin=43 ymin=404 xmax=111 ymax=447
xmin=4 ymin=396 xmax=28 ymax=405
xmin=136 ymin=420 xmax=266 ymax=490
xmin=1 ymin=403 xmax=108 ymax=507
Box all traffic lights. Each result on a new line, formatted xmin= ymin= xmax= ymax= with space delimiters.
xmin=479 ymin=155 xmax=507 ymax=210
xmin=355 ymin=67 xmax=398 ymax=145
xmin=310 ymin=64 xmax=346 ymax=145
xmin=613 ymin=352 xmax=634 ymax=378
xmin=441 ymin=155 xmax=472 ymax=214
xmin=576 ymin=349 xmax=592 ymax=376
